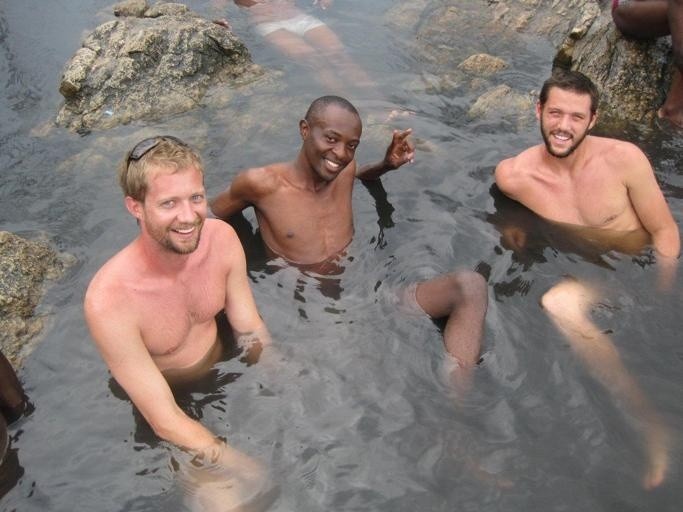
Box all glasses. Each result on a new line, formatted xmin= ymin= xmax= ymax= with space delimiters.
xmin=126 ymin=136 xmax=188 ymax=170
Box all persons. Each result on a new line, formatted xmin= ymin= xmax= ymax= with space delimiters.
xmin=80 ymin=130 xmax=288 ymax=511
xmin=232 ymin=0 xmax=414 ymax=124
xmin=493 ymin=69 xmax=682 ymax=491
xmin=609 ymin=0 xmax=682 ymax=128
xmin=207 ymin=93 xmax=489 ymax=409
